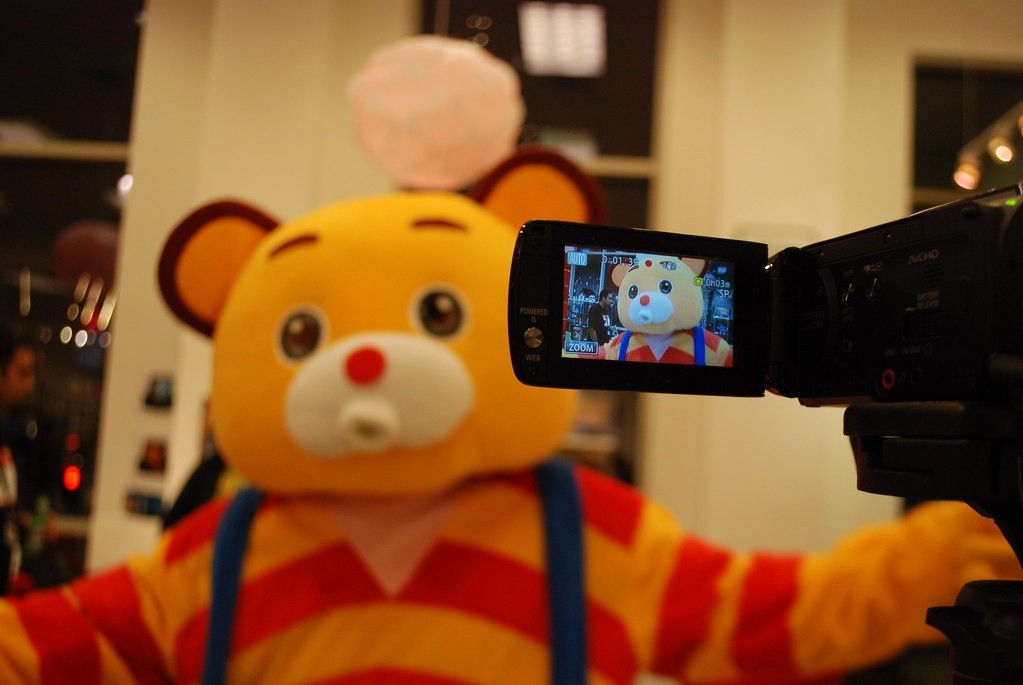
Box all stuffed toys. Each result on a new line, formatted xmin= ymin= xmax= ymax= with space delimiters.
xmin=576 ymin=255 xmax=732 ymax=367
xmin=0 ymin=149 xmax=1022 ymax=685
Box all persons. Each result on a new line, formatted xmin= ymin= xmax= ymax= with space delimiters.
xmin=0 ymin=331 xmax=91 ymax=601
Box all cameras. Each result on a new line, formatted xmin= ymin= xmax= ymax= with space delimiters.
xmin=509 ymin=180 xmax=1023 ymax=409
